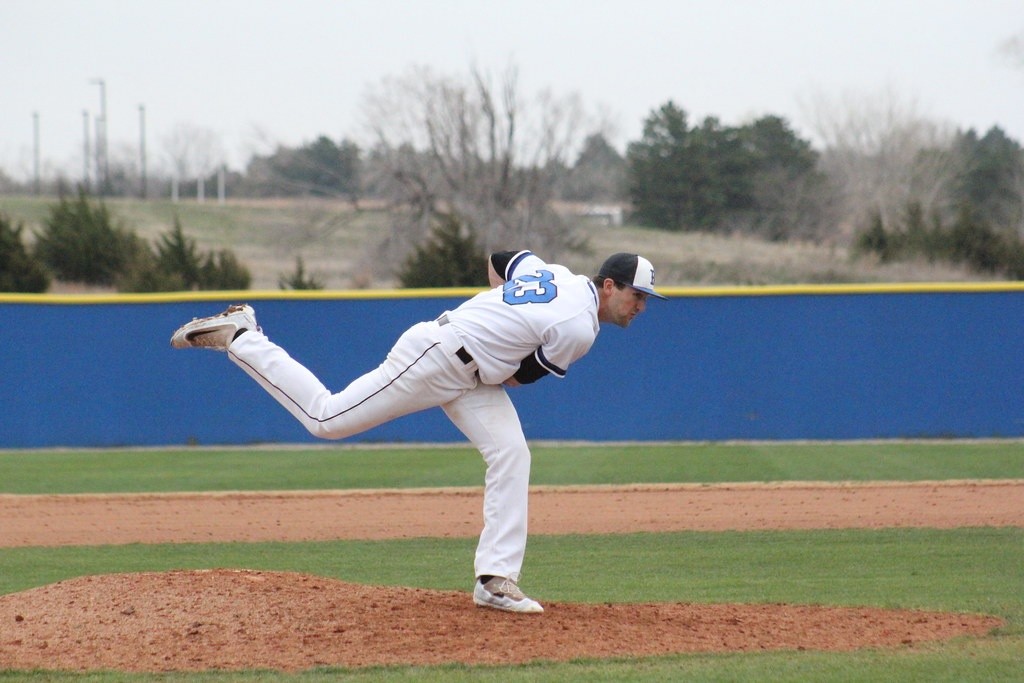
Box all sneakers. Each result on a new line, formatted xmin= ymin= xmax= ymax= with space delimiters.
xmin=473 ymin=571 xmax=544 ymax=613
xmin=170 ymin=304 xmax=257 ymax=351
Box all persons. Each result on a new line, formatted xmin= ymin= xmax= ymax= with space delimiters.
xmin=170 ymin=249 xmax=669 ymax=613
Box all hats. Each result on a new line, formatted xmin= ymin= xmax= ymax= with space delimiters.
xmin=598 ymin=252 xmax=669 ymax=301
